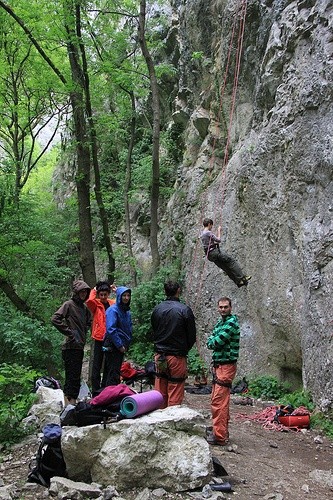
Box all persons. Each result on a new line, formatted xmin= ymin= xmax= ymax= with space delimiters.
xmin=151 ymin=281 xmax=196 ymax=409
xmin=200 ymin=217 xmax=252 ymax=288
xmin=104 ymin=286 xmax=133 ymax=387
xmin=85 ymin=285 xmax=117 ymax=398
xmin=51 ymin=280 xmax=93 ymax=406
xmin=205 ymin=297 xmax=241 ymax=445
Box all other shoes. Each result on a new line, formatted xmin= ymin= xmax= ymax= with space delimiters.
xmin=206 ymin=431 xmax=229 ymax=445
xmin=237 ymin=275 xmax=252 ymax=288
xmin=193 ymin=373 xmax=208 ymax=385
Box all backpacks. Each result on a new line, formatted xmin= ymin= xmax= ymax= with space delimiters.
xmin=27 ymin=435 xmax=65 ymax=487
